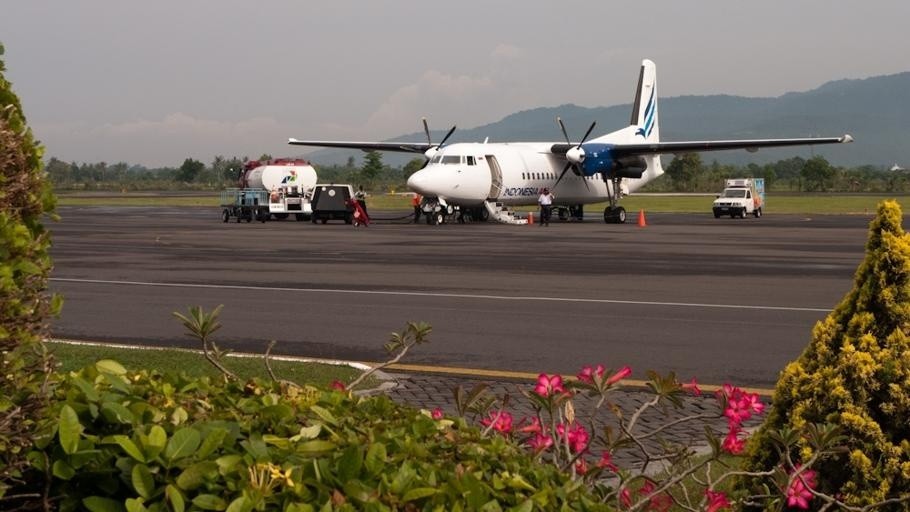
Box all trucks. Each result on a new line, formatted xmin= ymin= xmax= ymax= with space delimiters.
xmin=713 ymin=178 xmax=765 ymax=218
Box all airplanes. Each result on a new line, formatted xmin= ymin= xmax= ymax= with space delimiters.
xmin=289 ymin=59 xmax=850 ymax=224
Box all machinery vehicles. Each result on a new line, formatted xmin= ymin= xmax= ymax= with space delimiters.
xmin=239 ymin=159 xmax=318 ymax=221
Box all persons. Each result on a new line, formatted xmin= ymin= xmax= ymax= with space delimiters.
xmin=538 ymin=188 xmax=555 ymax=226
xmin=355 ymin=186 xmax=366 ymax=213
xmin=413 ymin=194 xmax=421 ymax=224
